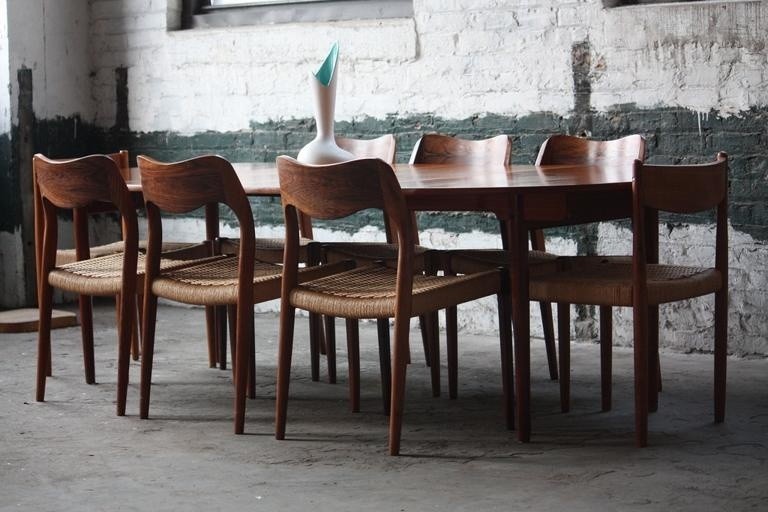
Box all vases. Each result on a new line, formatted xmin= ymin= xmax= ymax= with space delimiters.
xmin=295 ymin=40 xmax=354 ymax=166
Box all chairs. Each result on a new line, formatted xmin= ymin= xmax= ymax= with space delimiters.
xmin=263 ymin=152 xmax=517 ymax=453
xmin=31 ymin=152 xmax=221 ymax=418
xmin=33 ymin=150 xmax=220 ymax=376
xmin=319 ymin=136 xmax=560 ymax=405
xmin=502 ymin=150 xmax=727 ymax=451
xmin=214 ymin=136 xmax=419 ymax=404
xmin=129 ymin=153 xmax=367 ymax=441
xmin=439 ymin=134 xmax=667 ymax=418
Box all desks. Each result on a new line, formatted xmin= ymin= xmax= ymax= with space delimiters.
xmin=44 ymin=159 xmax=665 ymax=449
